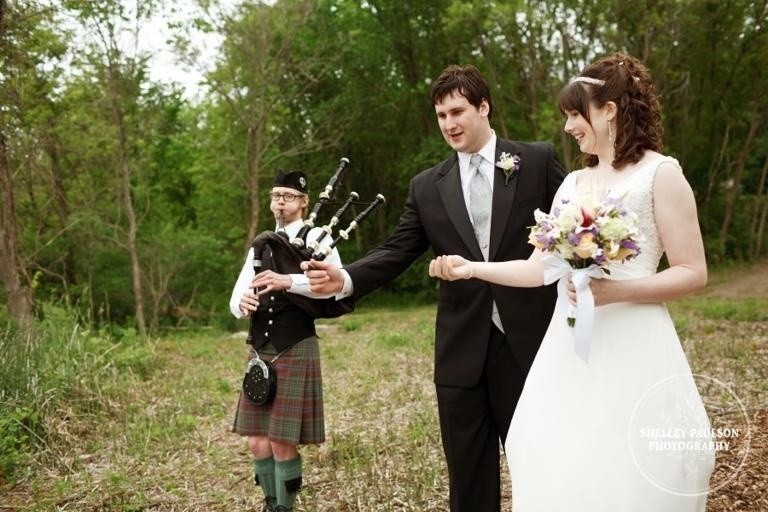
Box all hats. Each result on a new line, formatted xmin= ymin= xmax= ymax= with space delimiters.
xmin=272 ymin=170 xmax=308 ymax=193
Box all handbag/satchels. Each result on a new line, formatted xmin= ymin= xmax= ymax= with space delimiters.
xmin=243 ymin=359 xmax=276 ymax=405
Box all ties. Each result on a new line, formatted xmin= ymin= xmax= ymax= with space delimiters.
xmin=469 ymin=154 xmax=507 ymax=334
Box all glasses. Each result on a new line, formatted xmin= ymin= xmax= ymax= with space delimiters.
xmin=270 ymin=192 xmax=304 ymax=202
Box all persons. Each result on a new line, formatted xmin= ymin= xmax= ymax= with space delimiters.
xmin=427 ymin=51 xmax=718 ymax=512
xmin=228 ymin=170 xmax=343 ymax=511
xmin=299 ymin=60 xmax=570 ymax=512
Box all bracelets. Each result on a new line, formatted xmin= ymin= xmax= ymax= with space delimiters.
xmin=463 ymin=259 xmax=473 ymax=281
